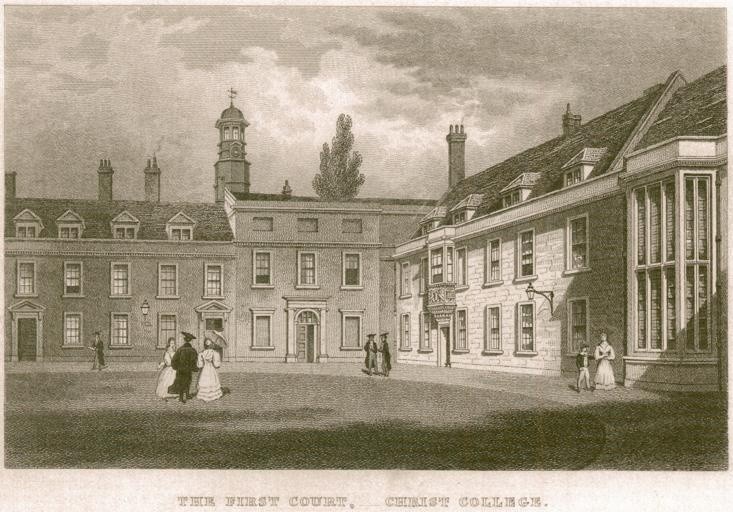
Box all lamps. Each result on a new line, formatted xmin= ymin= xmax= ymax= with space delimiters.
xmin=140 ymin=300 xmax=150 ymax=316
xmin=526 ymin=282 xmax=554 ymax=316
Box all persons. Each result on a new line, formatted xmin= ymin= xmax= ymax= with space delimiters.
xmin=576 ymin=342 xmax=595 ymax=394
xmin=196 ymin=337 xmax=223 ymax=403
xmin=593 ymin=333 xmax=616 ymax=392
xmin=168 ymin=331 xmax=199 ymax=403
xmin=157 ymin=337 xmax=179 ymax=401
xmin=364 ymin=333 xmax=379 ymax=376
xmin=87 ymin=331 xmax=106 ymax=372
xmin=377 ymin=332 xmax=391 ymax=377
